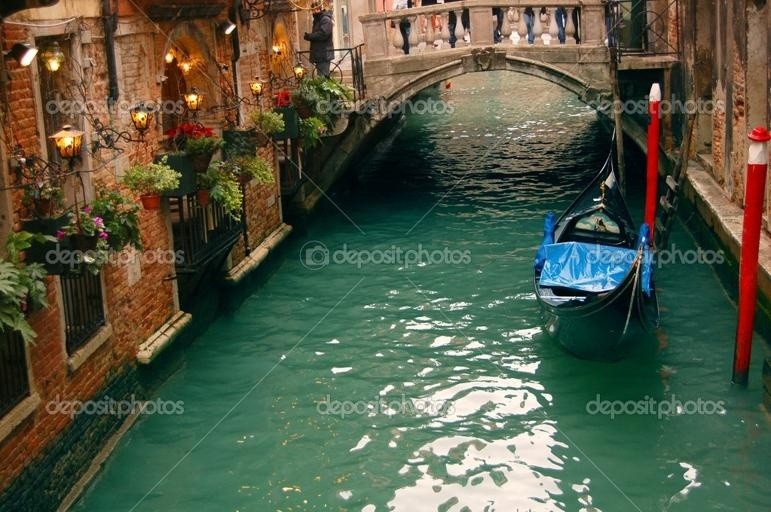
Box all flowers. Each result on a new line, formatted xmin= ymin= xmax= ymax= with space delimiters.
xmin=171 ymin=123 xmax=227 ymax=154
xmin=53 ymin=195 xmax=144 ymax=261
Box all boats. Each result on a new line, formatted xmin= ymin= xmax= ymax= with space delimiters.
xmin=534 ymin=126 xmax=658 ymax=362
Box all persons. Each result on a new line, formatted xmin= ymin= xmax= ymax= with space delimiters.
xmin=303 ymin=2 xmax=336 ymax=80
xmin=389 ymin=0 xmax=615 ymax=56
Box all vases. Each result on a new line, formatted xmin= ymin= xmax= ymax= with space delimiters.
xmin=68 ymin=233 xmax=96 ymax=257
xmin=191 ymin=154 xmax=210 ymax=170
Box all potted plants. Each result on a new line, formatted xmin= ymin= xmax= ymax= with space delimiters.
xmin=193 ymin=155 xmax=275 ymax=222
xmin=122 ymin=160 xmax=182 ymax=210
xmin=261 ymin=76 xmax=354 ymax=148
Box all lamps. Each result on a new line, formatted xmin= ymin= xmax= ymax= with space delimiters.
xmin=13 ymin=123 xmax=85 ymax=184
xmin=2 ymin=43 xmax=39 ymax=68
xmin=94 ymin=101 xmax=154 ymax=151
xmin=183 ymin=84 xmax=205 ymax=113
xmin=250 ymin=75 xmax=266 ymax=96
xmin=218 ymin=17 xmax=237 ymax=35
